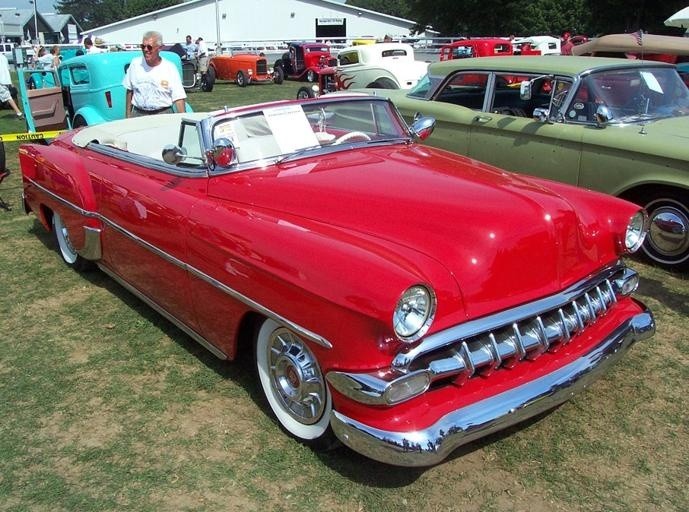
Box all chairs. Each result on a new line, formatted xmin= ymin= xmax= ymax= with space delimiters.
xmin=213 ymin=116 xmax=248 ymax=149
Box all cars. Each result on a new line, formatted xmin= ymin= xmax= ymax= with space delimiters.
xmin=318 ymin=54 xmax=688 ymax=267
xmin=17 ymin=45 xmax=286 ymax=133
xmin=17 ymin=95 xmax=656 ymax=469
xmin=275 ymin=42 xmax=430 ymax=98
xmin=439 ymin=33 xmax=689 ymax=103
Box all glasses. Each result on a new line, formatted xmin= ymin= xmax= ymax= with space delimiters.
xmin=140 ymin=44 xmax=157 ymax=51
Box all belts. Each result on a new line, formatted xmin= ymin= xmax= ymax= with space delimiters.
xmin=132 ymin=105 xmax=171 ymax=114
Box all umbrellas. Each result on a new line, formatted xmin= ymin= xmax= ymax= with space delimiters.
xmin=664 ymin=7 xmax=689 ymax=29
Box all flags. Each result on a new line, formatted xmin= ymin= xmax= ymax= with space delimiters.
xmin=631 ymin=29 xmax=644 ymax=47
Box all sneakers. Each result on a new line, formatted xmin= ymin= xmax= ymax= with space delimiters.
xmin=17 ymin=113 xmax=24 ymax=120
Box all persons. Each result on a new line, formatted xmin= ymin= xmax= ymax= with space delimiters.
xmin=84 ymin=38 xmax=104 ymax=55
xmin=0 ymin=53 xmax=25 ymax=121
xmin=30 ymin=44 xmax=62 ymax=73
xmin=182 ymin=35 xmax=199 ymax=63
xmin=192 ymin=36 xmax=209 ymax=61
xmin=76 ymin=50 xmax=84 ymax=56
xmin=561 ymin=32 xmax=574 ymax=56
xmin=121 ymin=30 xmax=189 ymax=119
